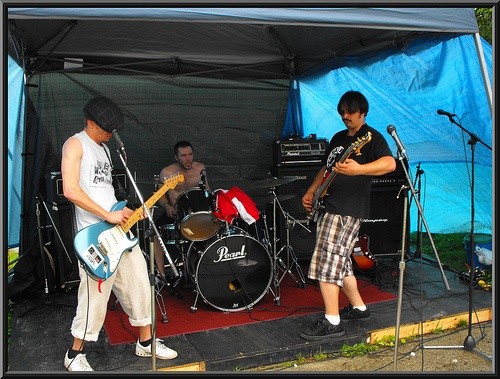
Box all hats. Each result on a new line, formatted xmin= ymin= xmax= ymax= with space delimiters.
xmin=85 ymin=96 xmax=124 ymax=132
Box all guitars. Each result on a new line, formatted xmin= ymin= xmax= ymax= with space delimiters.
xmin=305 ymin=131 xmax=372 ymax=228
xmin=70 ymin=172 xmax=184 ymax=283
xmin=351 ymin=233 xmax=378 ymax=272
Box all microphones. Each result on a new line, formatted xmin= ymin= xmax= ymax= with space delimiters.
xmin=200 ymin=171 xmax=206 ymax=186
xmin=387 ymin=125 xmax=409 ymax=163
xmin=109 ymin=124 xmax=125 ymax=151
xmin=437 ymin=109 xmax=457 ymax=118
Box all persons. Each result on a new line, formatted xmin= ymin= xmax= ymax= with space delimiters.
xmin=60 ymin=96 xmax=178 ymax=372
xmin=151 ymin=140 xmax=212 ymax=285
xmin=300 ymin=90 xmax=396 ymax=340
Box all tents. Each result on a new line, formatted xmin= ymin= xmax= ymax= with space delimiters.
xmin=8 ymin=7 xmax=494 ymax=356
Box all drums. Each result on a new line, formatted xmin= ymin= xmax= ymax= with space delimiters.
xmin=185 ymin=224 xmax=274 ymax=313
xmin=158 ymin=223 xmax=206 ymax=296
xmin=175 ymin=185 xmax=220 ymax=242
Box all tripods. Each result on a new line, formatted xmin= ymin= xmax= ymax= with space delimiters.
xmin=18 ymin=182 xmax=78 ymax=318
xmin=401 ymin=117 xmax=492 ymax=365
xmin=265 ymin=187 xmax=313 ymax=305
xmin=165 ymin=243 xmax=193 ymax=289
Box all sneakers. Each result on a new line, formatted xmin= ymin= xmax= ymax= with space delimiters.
xmin=135 ymin=338 xmax=179 ymax=359
xmin=64 ymin=349 xmax=94 ymax=371
xmin=340 ymin=303 xmax=371 ymax=321
xmin=301 ymin=315 xmax=346 ymax=340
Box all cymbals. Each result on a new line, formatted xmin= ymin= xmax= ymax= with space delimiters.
xmin=255 ymin=194 xmax=297 ymax=206
xmin=135 ymin=177 xmax=165 ymax=185
xmin=248 ymin=175 xmax=298 ymax=189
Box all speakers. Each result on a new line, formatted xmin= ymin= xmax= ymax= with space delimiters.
xmin=40 ymin=202 xmax=83 ymax=292
xmin=271 ymin=162 xmax=326 ymax=262
xmin=357 ymin=181 xmax=411 ymax=257
